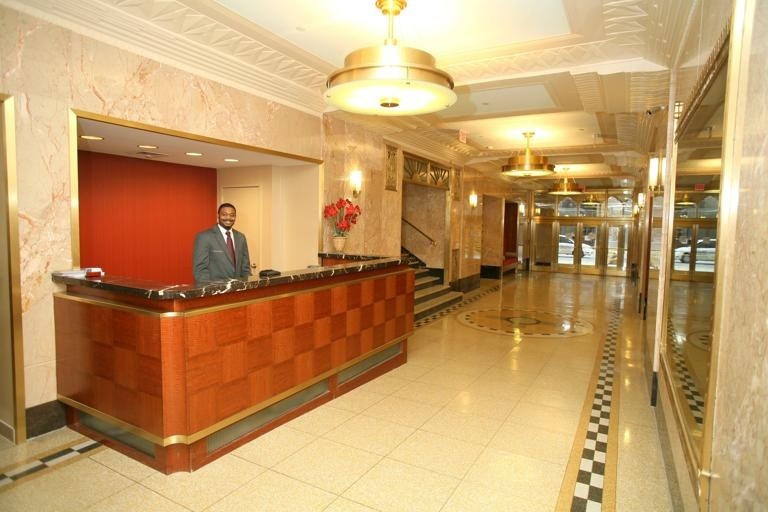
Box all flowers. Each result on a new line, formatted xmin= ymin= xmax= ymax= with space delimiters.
xmin=321 ymin=193 xmax=361 ymax=235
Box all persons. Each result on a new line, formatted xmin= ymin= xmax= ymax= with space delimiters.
xmin=192 ymin=202 xmax=252 ymax=283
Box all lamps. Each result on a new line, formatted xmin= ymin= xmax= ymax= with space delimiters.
xmin=671 ymin=99 xmax=684 ymax=120
xmin=468 ymin=188 xmax=477 ymax=209
xmin=499 ymin=126 xmax=558 ymax=180
xmin=675 ymin=193 xmax=696 ymax=207
xmin=350 ymin=170 xmax=361 ymax=198
xmin=548 ymin=167 xmax=584 ymax=196
xmin=582 ymin=191 xmax=600 ymax=206
xmin=646 ymin=156 xmax=659 ymax=193
xmin=319 ymin=0 xmax=458 ymax=117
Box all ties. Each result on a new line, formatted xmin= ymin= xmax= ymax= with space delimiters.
xmin=226 ymin=232 xmax=235 ymax=271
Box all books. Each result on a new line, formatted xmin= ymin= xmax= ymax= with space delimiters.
xmin=52 ymin=270 xmax=105 ymax=278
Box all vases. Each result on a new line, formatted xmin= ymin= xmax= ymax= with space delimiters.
xmin=332 ymin=237 xmax=347 ymax=252
